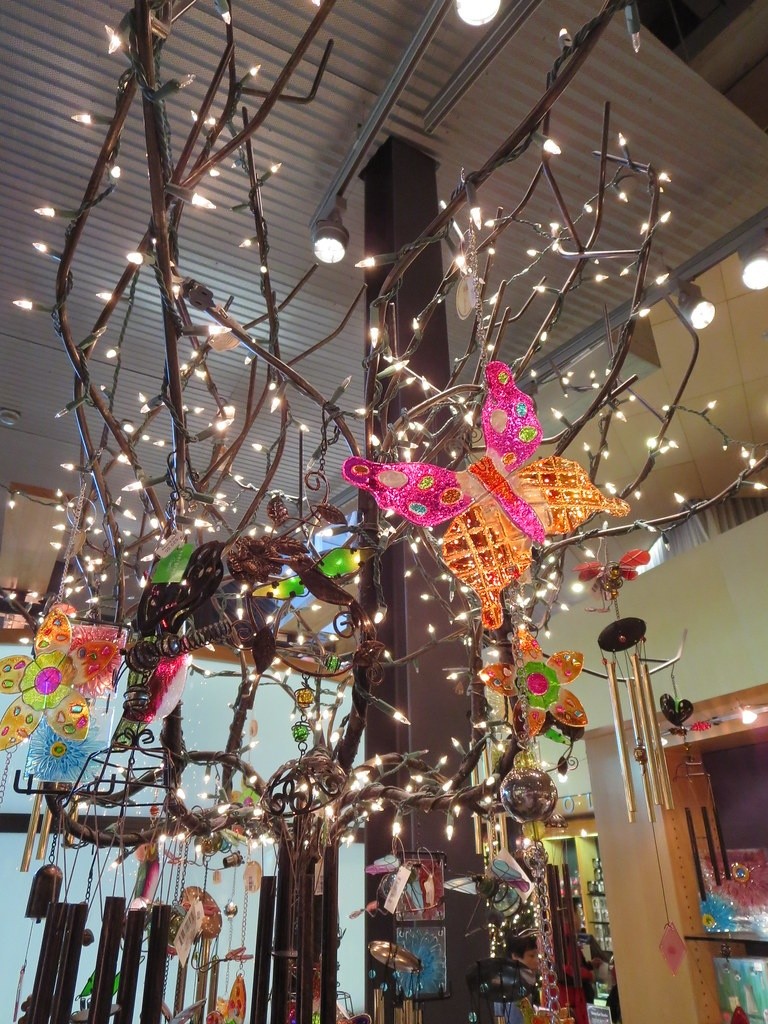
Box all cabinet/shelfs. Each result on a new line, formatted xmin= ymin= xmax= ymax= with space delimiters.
xmin=583 ymin=683 xmax=768 ymax=1024
xmin=542 ymin=833 xmax=611 ymax=955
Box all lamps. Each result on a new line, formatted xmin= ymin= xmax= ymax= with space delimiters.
xmin=312 ymin=196 xmax=349 ymax=263
xmin=665 ymin=265 xmax=715 ymax=329
xmin=738 ymin=232 xmax=768 ymax=290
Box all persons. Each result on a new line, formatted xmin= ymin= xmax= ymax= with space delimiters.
xmin=493 ymin=937 xmax=539 ymax=1024
xmin=545 ymin=896 xmax=612 ymax=1024
